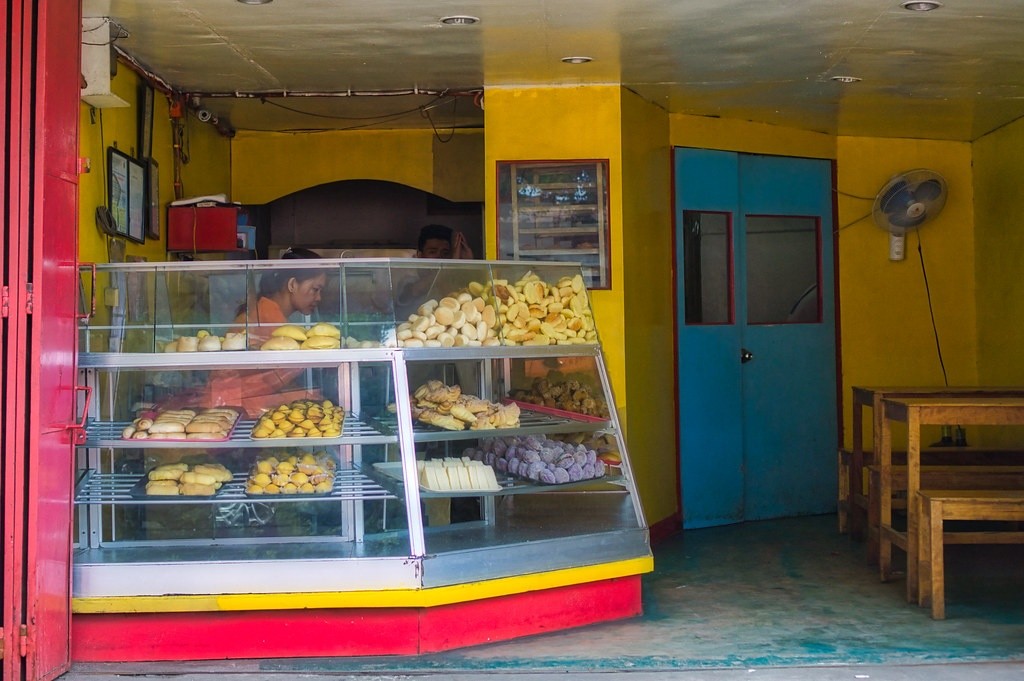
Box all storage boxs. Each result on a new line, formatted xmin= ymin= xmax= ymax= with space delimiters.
xmin=169 ymin=204 xmax=239 ymax=251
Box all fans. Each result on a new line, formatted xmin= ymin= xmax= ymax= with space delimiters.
xmin=871 ymin=169 xmax=948 ymax=260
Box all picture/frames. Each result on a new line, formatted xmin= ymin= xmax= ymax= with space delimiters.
xmin=106 ymin=145 xmax=160 ymax=245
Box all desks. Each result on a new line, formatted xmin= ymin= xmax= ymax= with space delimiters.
xmin=880 ymin=397 xmax=1024 ymax=603
xmin=851 ymin=385 xmax=1024 ymax=551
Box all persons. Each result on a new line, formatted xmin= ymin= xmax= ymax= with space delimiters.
xmin=384 ymin=224 xmax=480 ymax=410
xmin=198 ymin=251 xmax=329 ymax=538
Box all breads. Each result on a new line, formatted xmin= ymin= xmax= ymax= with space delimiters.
xmin=122 ymin=270 xmax=606 ymax=495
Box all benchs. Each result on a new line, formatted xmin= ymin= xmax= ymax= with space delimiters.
xmin=837 ymin=445 xmax=1024 ymax=537
xmin=917 ymin=489 xmax=1024 ymax=620
xmin=865 ymin=463 xmax=1024 ymax=575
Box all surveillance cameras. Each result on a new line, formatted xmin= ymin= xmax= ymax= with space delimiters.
xmin=196 ymin=110 xmax=211 ymax=122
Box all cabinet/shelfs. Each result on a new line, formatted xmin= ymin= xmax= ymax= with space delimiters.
xmin=68 ymin=260 xmax=654 ymax=660
xmin=511 ymin=162 xmax=606 ymax=288
